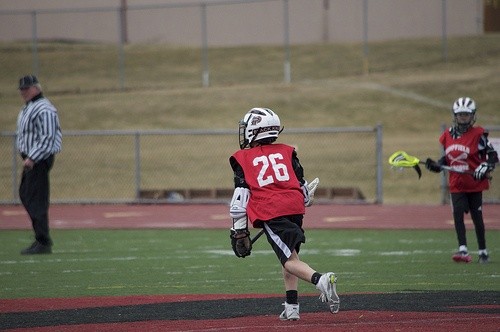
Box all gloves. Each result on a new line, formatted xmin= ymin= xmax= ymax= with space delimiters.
xmin=473 ymin=162 xmax=490 ymax=181
xmin=425 ymin=157 xmax=443 ymax=173
xmin=230 ymin=226 xmax=253 ymax=257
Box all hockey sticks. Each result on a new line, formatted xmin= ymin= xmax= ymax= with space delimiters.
xmin=250 ymin=178 xmax=320 ymax=245
xmin=388 ymin=150 xmax=493 ymax=181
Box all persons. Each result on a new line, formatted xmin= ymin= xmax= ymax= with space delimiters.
xmin=425 ymin=97 xmax=499 ymax=265
xmin=227 ymin=107 xmax=340 ymax=321
xmin=16 ymin=75 xmax=61 ymax=256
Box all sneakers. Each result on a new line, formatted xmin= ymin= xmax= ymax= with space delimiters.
xmin=452 ymin=250 xmax=472 ymax=263
xmin=21 ymin=242 xmax=53 ymax=255
xmin=477 ymin=254 xmax=489 ymax=264
xmin=279 ymin=301 xmax=302 ymax=321
xmin=316 ymin=272 xmax=341 ymax=314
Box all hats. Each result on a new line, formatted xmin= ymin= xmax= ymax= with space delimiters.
xmin=18 ymin=75 xmax=39 ymax=89
xmin=452 ymin=97 xmax=477 ymax=114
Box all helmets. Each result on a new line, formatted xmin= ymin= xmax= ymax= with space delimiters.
xmin=238 ymin=107 xmax=282 ymax=149
